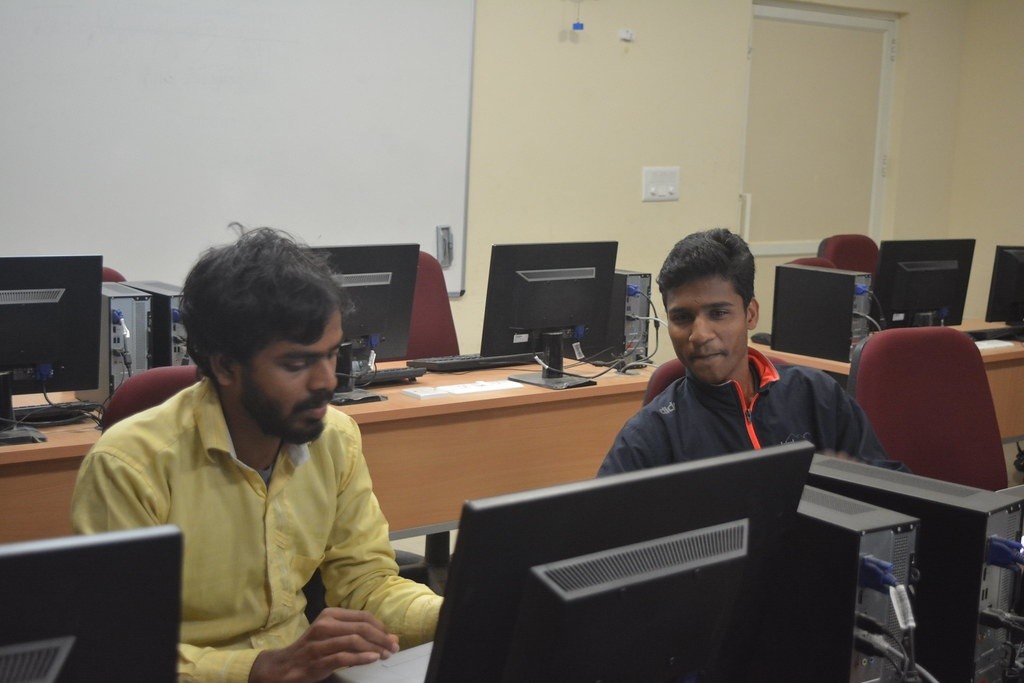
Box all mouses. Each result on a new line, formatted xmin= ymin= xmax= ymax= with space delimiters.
xmin=751 ymin=333 xmax=772 ymax=346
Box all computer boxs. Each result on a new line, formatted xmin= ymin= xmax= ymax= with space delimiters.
xmin=74 ymin=280 xmax=197 ymax=415
xmin=562 ymin=269 xmax=652 ymax=370
xmin=771 ymin=263 xmax=873 ymax=363
xmin=749 ymin=451 xmax=1023 ymax=683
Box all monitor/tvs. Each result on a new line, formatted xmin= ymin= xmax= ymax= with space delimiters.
xmin=985 ymin=246 xmax=1024 ymax=341
xmin=0 ymin=255 xmax=104 ymax=445
xmin=870 ymin=239 xmax=975 ymax=331
xmin=480 ymin=241 xmax=619 ymax=390
xmin=0 ymin=524 xmax=187 ymax=683
xmin=424 ymin=440 xmax=815 ymax=683
xmin=303 ymin=243 xmax=420 ymax=406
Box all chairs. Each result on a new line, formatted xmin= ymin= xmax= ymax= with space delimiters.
xmin=751 ymin=255 xmax=837 ymax=346
xmin=375 ymin=250 xmax=460 ymax=363
xmin=818 ymin=234 xmax=879 ymax=289
xmin=846 ymin=325 xmax=1008 ymax=492
xmin=100 ymin=364 xmax=199 ymax=435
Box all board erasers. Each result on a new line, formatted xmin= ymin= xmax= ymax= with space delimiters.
xmin=436 ymin=225 xmax=453 ymax=269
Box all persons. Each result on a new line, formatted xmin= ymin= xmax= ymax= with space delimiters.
xmin=65 ymin=222 xmax=444 ymax=683
xmin=595 ymin=226 xmax=890 ymax=472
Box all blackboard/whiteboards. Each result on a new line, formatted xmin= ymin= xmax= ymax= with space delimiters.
xmin=0 ymin=0 xmax=477 ymax=299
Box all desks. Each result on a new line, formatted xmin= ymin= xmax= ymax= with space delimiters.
xmin=0 ymin=351 xmax=668 ymax=573
xmin=748 ymin=316 xmax=1024 ymax=447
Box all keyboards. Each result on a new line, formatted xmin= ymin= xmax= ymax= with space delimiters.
xmin=966 ymin=329 xmax=1007 ymax=341
xmin=353 ymin=367 xmax=427 ymax=386
xmin=407 ymin=352 xmax=543 ymax=372
xmin=13 ymin=401 xmax=96 ymax=422
